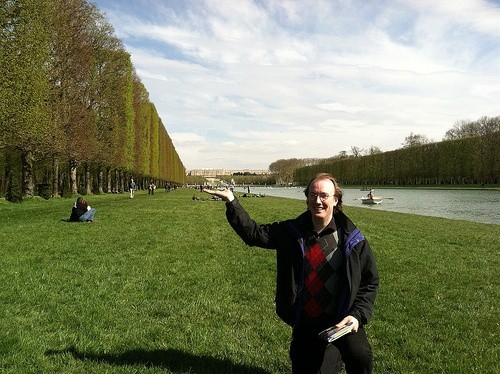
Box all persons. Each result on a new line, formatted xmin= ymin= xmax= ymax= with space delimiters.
xmin=70 ymin=179 xmax=233 ymax=222
xmin=201 ymin=173 xmax=379 ymax=374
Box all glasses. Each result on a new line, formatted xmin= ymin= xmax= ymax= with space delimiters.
xmin=308 ymin=192 xmax=335 ymax=201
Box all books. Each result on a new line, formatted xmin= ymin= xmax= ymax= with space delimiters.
xmin=320 ymin=321 xmax=352 ymax=342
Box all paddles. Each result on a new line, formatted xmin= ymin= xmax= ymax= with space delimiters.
xmin=354 ymin=198 xmax=394 ymax=201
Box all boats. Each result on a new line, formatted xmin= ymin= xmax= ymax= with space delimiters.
xmin=361 ymin=196 xmax=382 ymax=204
